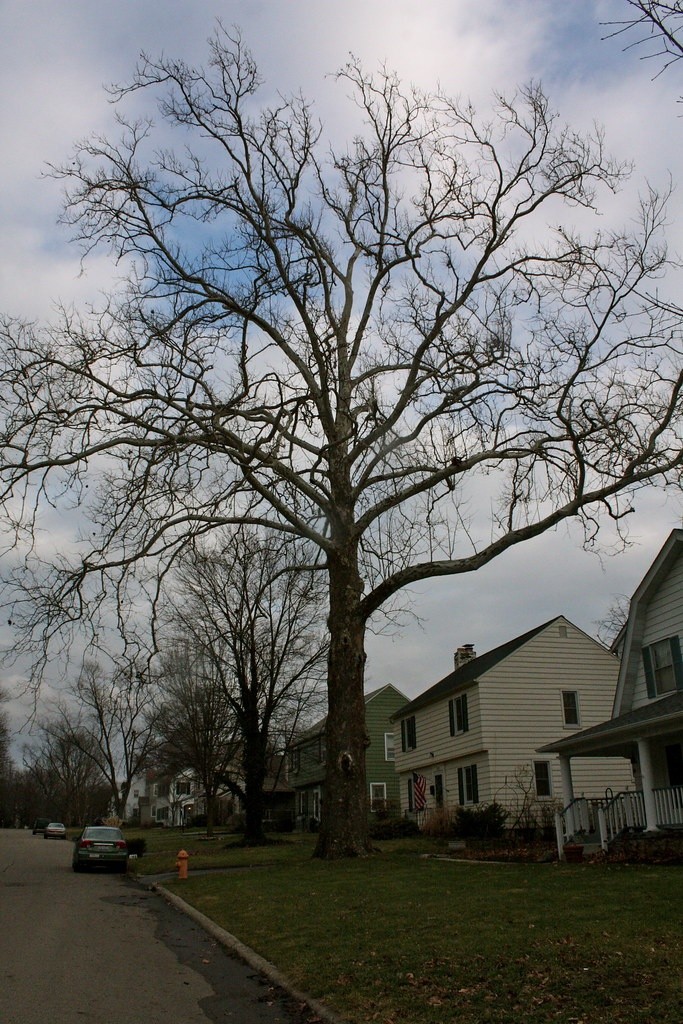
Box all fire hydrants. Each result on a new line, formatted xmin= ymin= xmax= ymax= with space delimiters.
xmin=175 ymin=849 xmax=188 ymax=878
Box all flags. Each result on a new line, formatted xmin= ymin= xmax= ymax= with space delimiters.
xmin=412 ymin=772 xmax=428 ymax=811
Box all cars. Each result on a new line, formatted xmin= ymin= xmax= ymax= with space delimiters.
xmin=72 ymin=826 xmax=129 ymax=875
xmin=44 ymin=823 xmax=66 ymax=840
xmin=32 ymin=818 xmax=52 ymax=835
